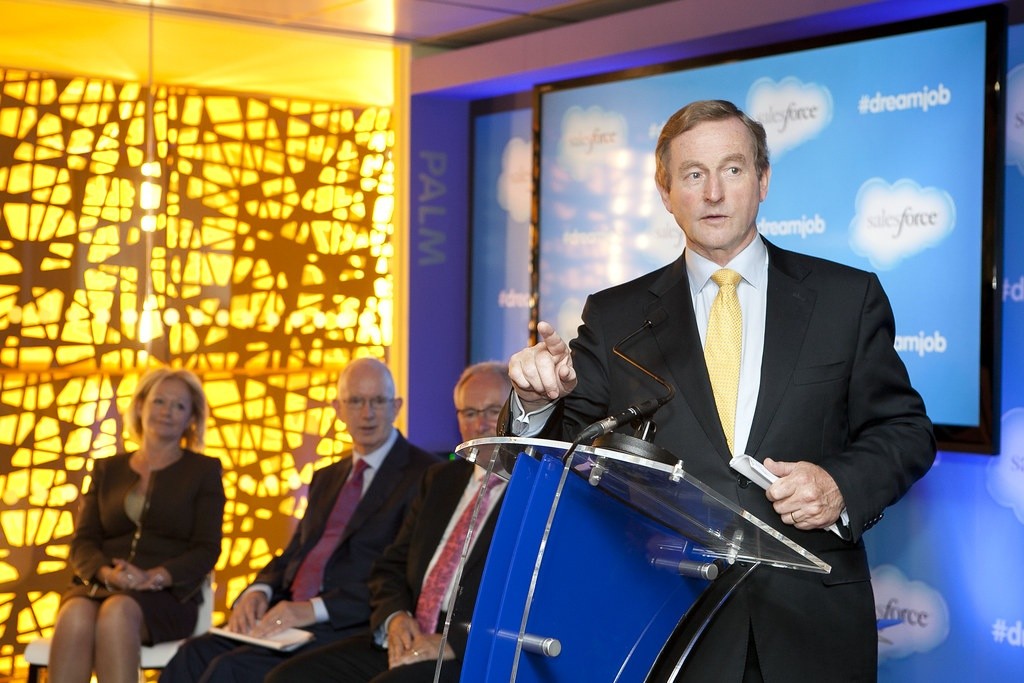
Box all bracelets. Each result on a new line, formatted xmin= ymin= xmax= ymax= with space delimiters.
xmin=105 ymin=569 xmax=115 ymax=592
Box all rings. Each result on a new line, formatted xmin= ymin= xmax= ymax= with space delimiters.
xmin=127 ymin=573 xmax=133 ymax=579
xmin=791 ymin=512 xmax=798 ymax=523
xmin=276 ymin=620 xmax=282 ymax=625
xmin=412 ymin=649 xmax=419 ymax=656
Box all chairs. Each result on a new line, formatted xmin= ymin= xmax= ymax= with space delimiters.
xmin=23 ymin=569 xmax=213 ymax=683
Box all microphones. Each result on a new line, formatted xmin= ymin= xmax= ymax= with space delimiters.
xmin=578 ymin=308 xmax=676 ymax=440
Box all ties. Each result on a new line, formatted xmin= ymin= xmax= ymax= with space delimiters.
xmin=704 ymin=267 xmax=743 ymax=460
xmin=290 ymin=459 xmax=370 ymax=602
xmin=416 ymin=473 xmax=505 ymax=635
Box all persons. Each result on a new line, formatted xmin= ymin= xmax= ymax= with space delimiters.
xmin=153 ymin=357 xmax=445 ymax=683
xmin=496 ymin=100 xmax=939 ymax=683
xmin=267 ymin=361 xmax=514 ymax=683
xmin=48 ymin=368 xmax=227 ymax=682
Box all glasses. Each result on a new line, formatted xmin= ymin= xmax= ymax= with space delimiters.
xmin=455 ymin=403 xmax=501 ymax=424
xmin=335 ymin=394 xmax=395 ymax=409
